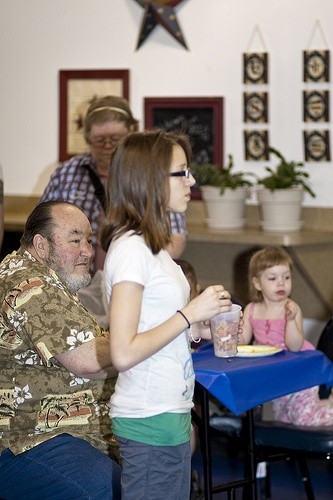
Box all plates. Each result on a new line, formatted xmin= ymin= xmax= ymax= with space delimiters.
xmin=234 ymin=345 xmax=283 ymax=358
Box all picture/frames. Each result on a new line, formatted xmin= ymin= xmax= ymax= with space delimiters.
xmin=143 ymin=96 xmax=224 ymax=200
xmin=58 ymin=68 xmax=131 ymax=163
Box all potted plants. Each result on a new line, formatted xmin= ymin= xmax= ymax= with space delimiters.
xmin=255 ymin=147 xmax=317 ymax=232
xmin=190 ymin=153 xmax=261 ymax=227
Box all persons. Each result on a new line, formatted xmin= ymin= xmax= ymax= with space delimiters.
xmin=1 ymin=200 xmax=120 ymax=500
xmin=236 ymin=245 xmax=333 ymax=427
xmin=43 ymin=95 xmax=188 ymax=262
xmin=173 ymin=258 xmax=201 ymax=301
xmin=99 ymin=130 xmax=243 ymax=500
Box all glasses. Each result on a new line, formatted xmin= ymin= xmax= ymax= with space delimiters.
xmin=170 ymin=167 xmax=196 ymax=178
xmin=89 ymin=137 xmax=122 ymax=147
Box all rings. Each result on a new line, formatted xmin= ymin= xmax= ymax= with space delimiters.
xmin=219 ymin=291 xmax=224 ymax=298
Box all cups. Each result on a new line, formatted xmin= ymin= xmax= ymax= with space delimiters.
xmin=209 ymin=303 xmax=242 ymax=358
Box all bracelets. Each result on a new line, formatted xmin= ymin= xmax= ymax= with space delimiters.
xmin=176 ymin=310 xmax=191 ymax=331
xmin=188 ymin=324 xmax=201 ymax=343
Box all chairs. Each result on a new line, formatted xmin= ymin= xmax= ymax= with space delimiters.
xmin=208 ymin=317 xmax=333 ymax=500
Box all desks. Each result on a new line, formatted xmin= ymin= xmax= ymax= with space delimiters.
xmin=0 ymin=199 xmax=333 ymax=318
xmin=190 ymin=337 xmax=333 ymax=500
